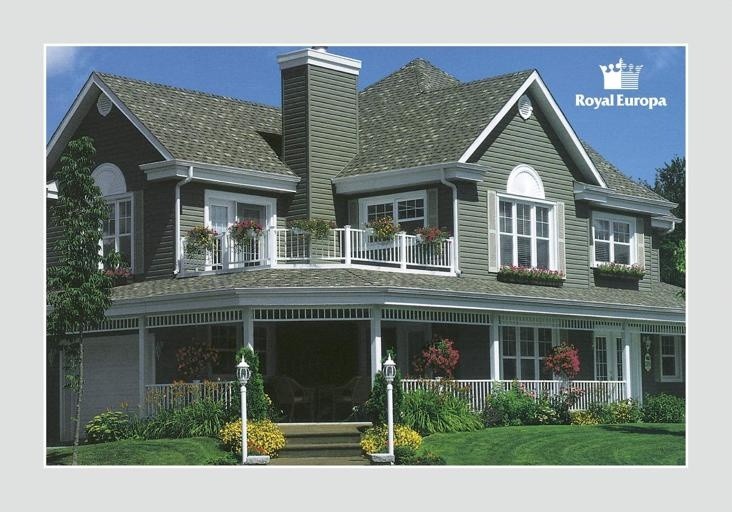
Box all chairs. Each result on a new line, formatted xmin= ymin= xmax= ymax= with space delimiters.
xmin=270 ymin=375 xmax=363 ymax=423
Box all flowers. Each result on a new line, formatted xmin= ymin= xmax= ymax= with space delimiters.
xmin=598 ymin=260 xmax=643 ymax=274
xmin=544 ymin=341 xmax=581 ymax=378
xmin=181 ymin=213 xmax=450 ymax=256
xmin=501 ymin=262 xmax=566 ymax=281
xmin=175 ymin=339 xmax=221 ymax=376
xmin=104 ymin=265 xmax=133 ymax=278
xmin=411 ymin=335 xmax=459 ymax=378
